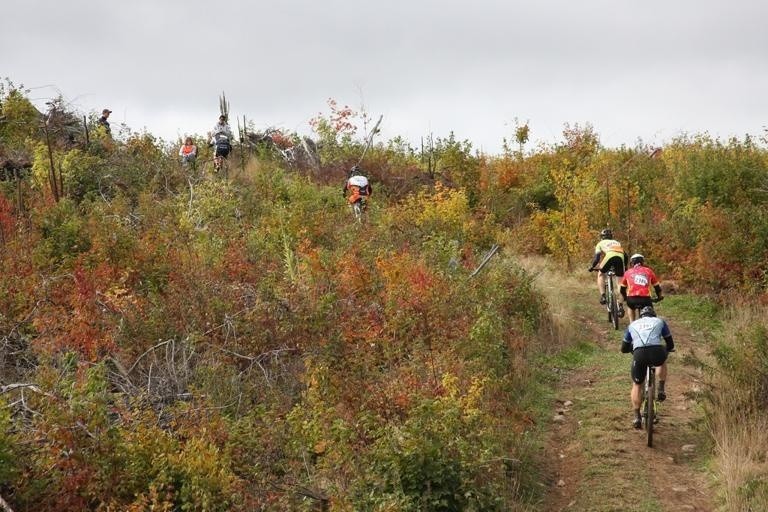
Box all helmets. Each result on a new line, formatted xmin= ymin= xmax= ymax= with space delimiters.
xmin=630 ymin=254 xmax=645 ymax=267
xmin=640 ymin=306 xmax=656 ymax=317
xmin=599 ymin=229 xmax=612 ymax=240
xmin=351 ymin=166 xmax=359 ymax=176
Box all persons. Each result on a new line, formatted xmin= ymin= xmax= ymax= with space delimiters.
xmin=619 ymin=307 xmax=675 ymax=428
xmin=179 ymin=138 xmax=197 ymax=166
xmin=208 ymin=114 xmax=234 ymax=172
xmin=620 ymin=253 xmax=664 ymax=324
xmin=97 ymin=109 xmax=112 ymax=134
xmin=342 ymin=165 xmax=372 ymax=219
xmin=588 ymin=229 xmax=629 ymax=304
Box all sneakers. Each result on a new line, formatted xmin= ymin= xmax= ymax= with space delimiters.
xmin=600 ymin=297 xmax=606 ymax=304
xmin=617 ymin=308 xmax=625 ymax=318
xmin=658 ymin=393 xmax=666 ymax=401
xmin=633 ymin=418 xmax=641 ymax=428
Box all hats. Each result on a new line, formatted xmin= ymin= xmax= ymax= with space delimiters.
xmin=103 ymin=109 xmax=111 ymax=112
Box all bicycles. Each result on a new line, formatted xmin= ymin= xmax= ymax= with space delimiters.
xmin=631 ymin=345 xmax=675 ymax=447
xmin=590 ymin=267 xmax=624 ymax=331
